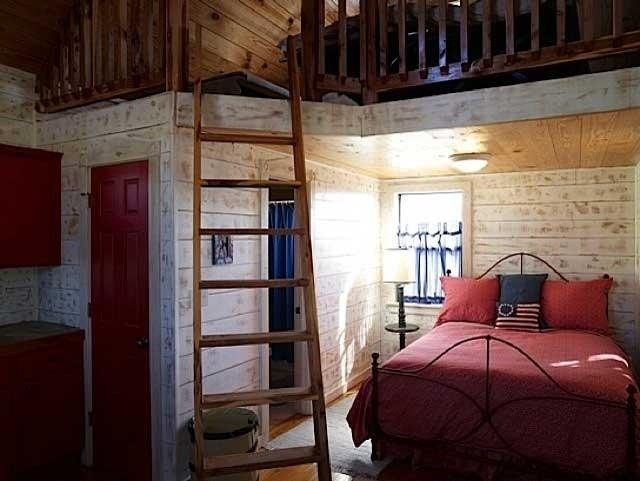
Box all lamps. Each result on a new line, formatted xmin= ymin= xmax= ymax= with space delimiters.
xmin=448 ymin=152 xmax=492 ymax=173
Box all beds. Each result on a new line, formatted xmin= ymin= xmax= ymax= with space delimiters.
xmin=371 ymin=251 xmax=638 ymax=480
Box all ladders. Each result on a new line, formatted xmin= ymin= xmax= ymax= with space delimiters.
xmin=192 ymin=24 xmax=335 ymax=481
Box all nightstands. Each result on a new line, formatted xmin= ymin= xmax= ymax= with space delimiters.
xmin=384 ymin=249 xmax=420 ymax=350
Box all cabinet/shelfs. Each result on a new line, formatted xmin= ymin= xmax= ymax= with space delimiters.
xmin=0 ymin=142 xmax=64 ymax=266
xmin=0 ymin=320 xmax=86 ymax=427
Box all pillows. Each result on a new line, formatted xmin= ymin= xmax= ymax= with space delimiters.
xmin=542 ymin=277 xmax=613 ymax=332
xmin=434 ymin=276 xmax=499 ymax=327
xmin=495 ymin=274 xmax=548 ymax=331
